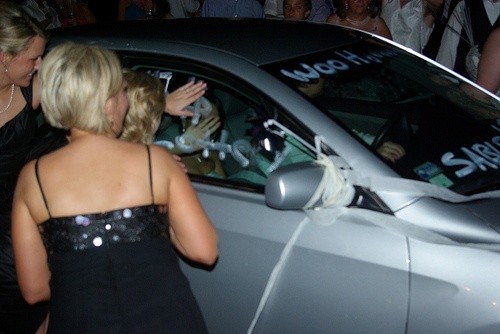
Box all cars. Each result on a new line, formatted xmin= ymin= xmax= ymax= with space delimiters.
xmin=44 ymin=18 xmax=499 ymax=332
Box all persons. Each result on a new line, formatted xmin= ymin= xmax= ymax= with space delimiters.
xmin=0 ymin=1 xmax=500 ymax=334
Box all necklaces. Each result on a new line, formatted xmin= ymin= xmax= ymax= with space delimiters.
xmin=0 ymin=83 xmax=15 ymax=114
xmin=348 ymin=13 xmax=367 ymax=20
